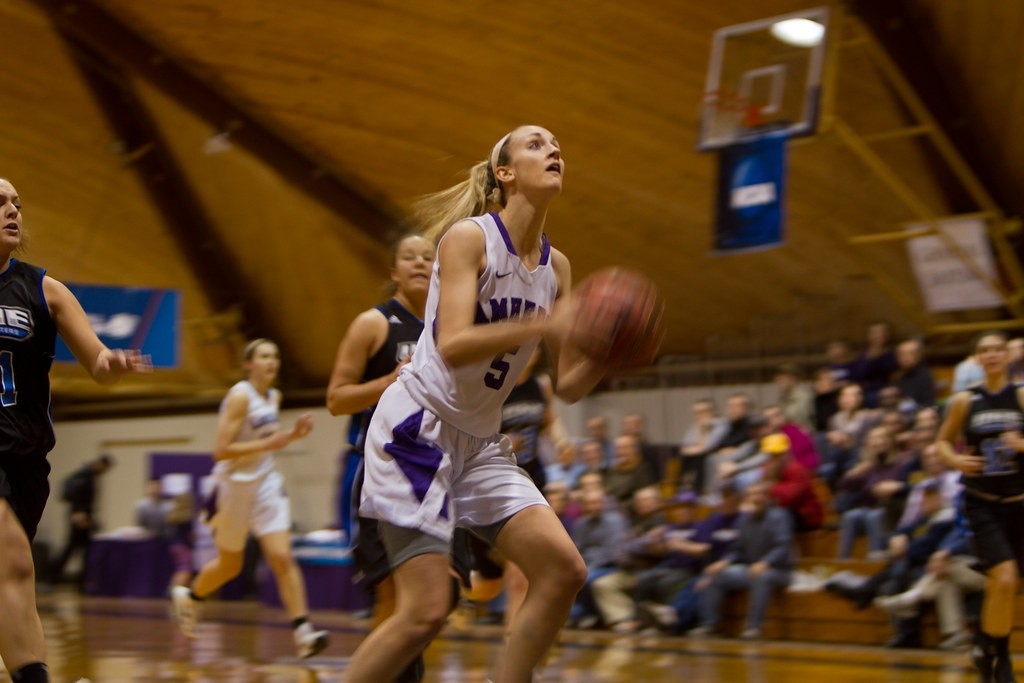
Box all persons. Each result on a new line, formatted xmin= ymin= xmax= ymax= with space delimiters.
xmin=0 ymin=176 xmax=331 ymax=683
xmin=328 ymin=124 xmax=608 ymax=683
xmin=545 ymin=323 xmax=1024 ymax=683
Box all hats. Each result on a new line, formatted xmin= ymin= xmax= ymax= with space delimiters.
xmin=761 ymin=432 xmax=792 ymax=455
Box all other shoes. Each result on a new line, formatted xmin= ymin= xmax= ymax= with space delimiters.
xmin=169 ymin=586 xmax=208 ymax=640
xmin=934 ymin=628 xmax=967 ymax=648
xmin=820 ymin=577 xmax=876 ymax=609
xmin=879 ymin=586 xmax=920 ymax=615
xmin=880 ymin=618 xmax=928 ymax=652
xmin=291 ymin=618 xmax=332 ymax=662
xmin=568 ymin=606 xmax=769 ymax=640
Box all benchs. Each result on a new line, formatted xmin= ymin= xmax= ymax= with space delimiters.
xmin=548 ymin=385 xmax=1024 ymax=652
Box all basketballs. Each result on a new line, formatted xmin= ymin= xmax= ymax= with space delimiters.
xmin=574 ymin=264 xmax=667 ymax=371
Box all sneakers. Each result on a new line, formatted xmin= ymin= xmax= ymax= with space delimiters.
xmin=977 ymin=656 xmax=1013 ymax=682
